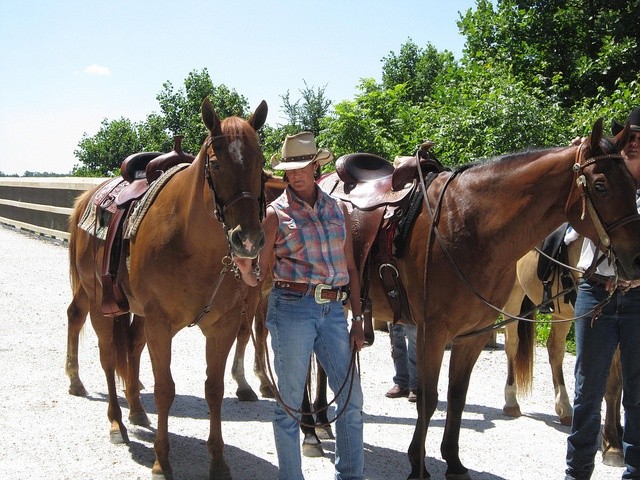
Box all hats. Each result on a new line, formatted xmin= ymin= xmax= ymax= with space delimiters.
xmin=611 ymin=108 xmax=640 ymax=138
xmin=271 ymin=132 xmax=333 ymax=170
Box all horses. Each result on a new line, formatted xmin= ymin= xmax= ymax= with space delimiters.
xmin=301 ymin=116 xmax=640 ymax=480
xmin=503 ymin=234 xmax=627 ymax=467
xmin=65 ymin=172 xmax=289 ymax=400
xmin=76 ymin=98 xmax=269 ymax=480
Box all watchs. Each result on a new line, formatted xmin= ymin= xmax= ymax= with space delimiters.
xmin=351 ymin=316 xmax=363 ymax=321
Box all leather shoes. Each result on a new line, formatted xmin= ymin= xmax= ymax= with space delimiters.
xmin=409 ymin=390 xmax=417 ymax=402
xmin=386 ymin=385 xmax=408 ymax=397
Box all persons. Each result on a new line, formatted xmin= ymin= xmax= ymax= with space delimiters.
xmin=233 ymin=132 xmax=367 ymax=480
xmin=565 ymin=110 xmax=640 ymax=479
xmin=386 ymin=324 xmax=418 ymax=402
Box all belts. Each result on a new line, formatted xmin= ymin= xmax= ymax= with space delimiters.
xmin=276 ymin=282 xmax=350 ymax=304
